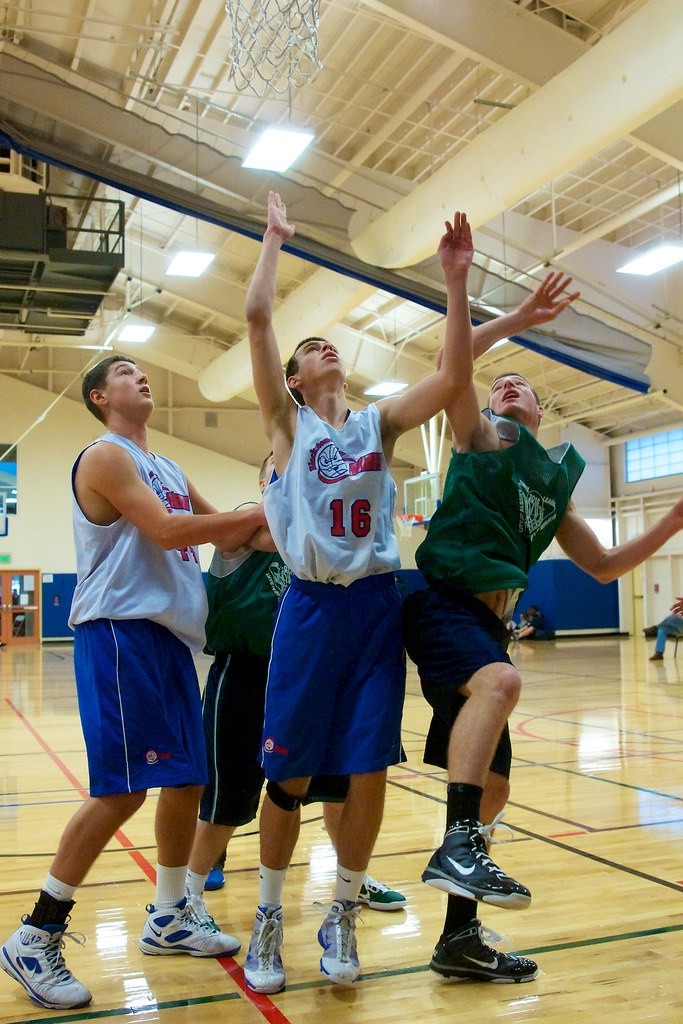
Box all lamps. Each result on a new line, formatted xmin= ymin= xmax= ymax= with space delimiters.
xmin=241 ymin=9 xmax=315 ymax=172
xmin=165 ymin=96 xmax=216 ymax=277
xmin=616 ymin=170 xmax=683 ymax=277
xmin=116 ymin=200 xmax=155 ymax=343
xmin=362 ymin=310 xmax=408 ymax=397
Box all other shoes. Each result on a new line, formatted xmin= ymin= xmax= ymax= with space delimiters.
xmin=650 ymin=652 xmax=664 ymax=660
xmin=642 ymin=625 xmax=658 ymax=636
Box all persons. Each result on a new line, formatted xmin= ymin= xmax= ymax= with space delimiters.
xmin=507 ymin=606 xmax=556 ymax=641
xmin=12 ymin=588 xmax=19 ymax=605
xmin=244 ymin=191 xmax=474 ymax=993
xmin=643 ymin=615 xmax=683 ymax=660
xmin=0 ymin=357 xmax=267 ymax=1009
xmin=409 ymin=273 xmax=683 ymax=983
xmin=182 ymin=450 xmax=407 ymax=934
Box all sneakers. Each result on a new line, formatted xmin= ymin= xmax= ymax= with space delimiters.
xmin=185 ymin=894 xmax=222 ymax=933
xmin=311 ymin=900 xmax=365 ymax=984
xmin=422 ymin=812 xmax=531 ymax=910
xmin=243 ymin=905 xmax=286 ymax=993
xmin=0 ymin=914 xmax=93 ymax=1009
xmin=429 ymin=919 xmax=539 ymax=983
xmin=357 ymin=875 xmax=407 ymax=910
xmin=205 ymin=868 xmax=226 ymax=891
xmin=138 ymin=898 xmax=240 ymax=957
